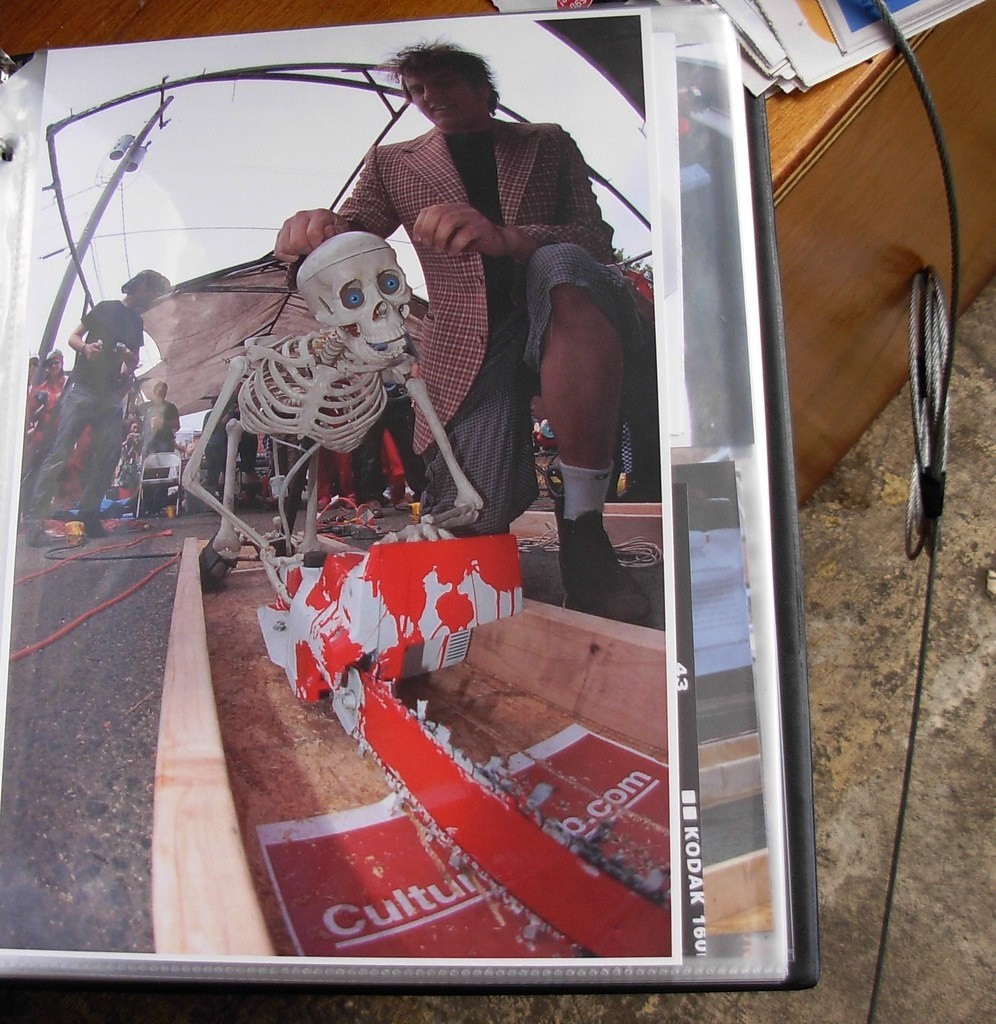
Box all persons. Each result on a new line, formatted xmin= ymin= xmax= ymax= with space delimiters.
xmin=17 ymin=44 xmax=663 ymax=627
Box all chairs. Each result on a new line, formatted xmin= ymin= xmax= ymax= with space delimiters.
xmin=136 ymin=451 xmax=182 ymax=519
